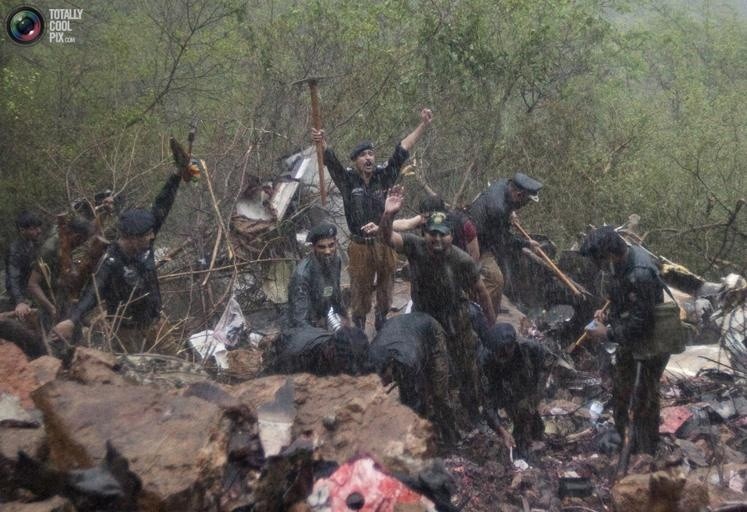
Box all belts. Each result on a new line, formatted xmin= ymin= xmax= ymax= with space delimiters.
xmin=351 ymin=234 xmax=392 ymax=243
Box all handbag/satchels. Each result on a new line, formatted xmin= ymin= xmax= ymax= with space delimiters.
xmin=629 ymin=299 xmax=686 ymax=359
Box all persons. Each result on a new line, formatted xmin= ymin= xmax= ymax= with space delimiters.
xmin=478 ymin=323 xmax=557 ymax=452
xmin=464 ymin=174 xmax=544 ymax=320
xmin=5 ymin=155 xmax=197 ymax=360
xmin=266 ymin=183 xmax=496 ymax=449
xmin=311 ymin=108 xmax=434 ymax=334
xmin=579 ymin=230 xmax=669 ymax=475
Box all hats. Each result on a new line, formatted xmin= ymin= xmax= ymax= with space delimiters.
xmin=68 ymin=214 xmax=96 ymax=240
xmin=306 ymin=222 xmax=339 ymax=243
xmin=15 ymin=211 xmax=43 ymax=226
xmin=578 ymin=225 xmax=616 ymax=257
xmin=482 ymin=321 xmax=518 ymax=354
xmin=419 ymin=193 xmax=445 ymax=214
xmin=332 ymin=325 xmax=370 ymax=365
xmin=511 ymin=173 xmax=544 ymax=203
xmin=95 ymin=190 xmax=113 ymax=201
xmin=350 ymin=143 xmax=375 ymax=161
xmin=116 ymin=209 xmax=157 ymax=239
xmin=422 ymin=211 xmax=455 ymax=236
